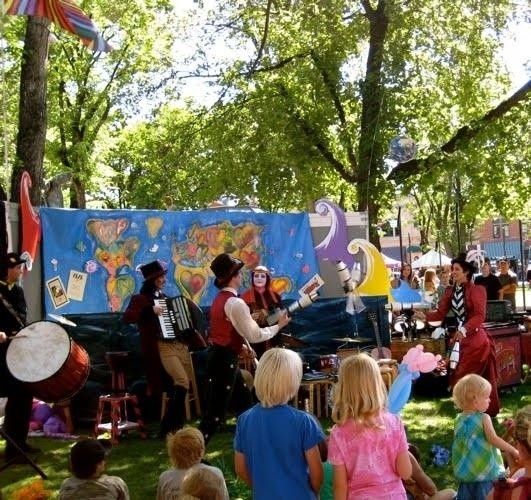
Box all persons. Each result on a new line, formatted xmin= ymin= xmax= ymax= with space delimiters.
xmin=1 ymin=252 xmax=530 ymax=500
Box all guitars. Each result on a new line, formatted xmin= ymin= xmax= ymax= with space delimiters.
xmin=366 ymin=311 xmax=392 ymax=360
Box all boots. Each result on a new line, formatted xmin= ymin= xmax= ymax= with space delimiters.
xmin=161 ymin=384 xmax=188 ymax=435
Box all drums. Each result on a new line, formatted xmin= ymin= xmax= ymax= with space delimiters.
xmin=5 ymin=320 xmax=91 ymax=402
xmin=319 ymin=354 xmax=339 ymax=371
xmin=337 ymin=349 xmax=358 ymax=359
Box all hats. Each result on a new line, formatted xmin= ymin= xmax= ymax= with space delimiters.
xmin=209 ymin=252 xmax=245 ymax=289
xmin=138 ymin=259 xmax=167 ymax=281
xmin=499 ymin=256 xmax=511 ymax=263
xmin=451 ymin=252 xmax=478 ymax=272
xmin=8 ymin=252 xmax=26 ymax=267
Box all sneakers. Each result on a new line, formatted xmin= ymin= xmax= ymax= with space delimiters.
xmin=4 ymin=443 xmax=41 ymax=456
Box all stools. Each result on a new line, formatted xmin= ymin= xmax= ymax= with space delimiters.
xmin=158 ymin=351 xmax=402 ymax=419
xmin=92 ymin=391 xmax=146 ymax=448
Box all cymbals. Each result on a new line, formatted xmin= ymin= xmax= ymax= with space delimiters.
xmin=276 ymin=333 xmax=310 ymax=348
xmin=332 ymin=336 xmax=369 ymax=343
